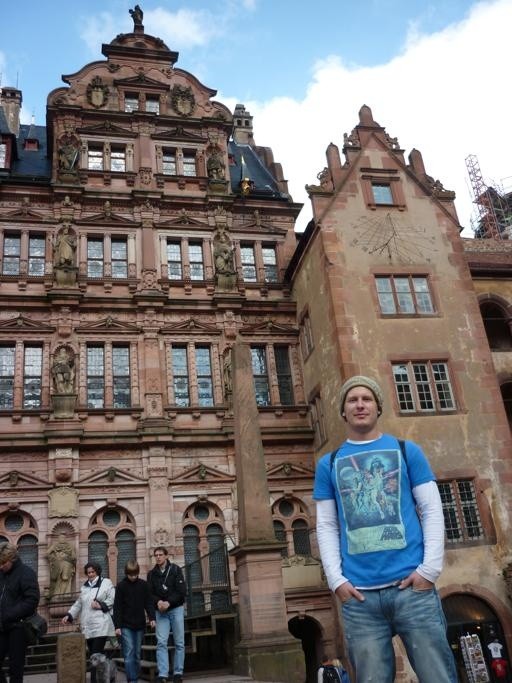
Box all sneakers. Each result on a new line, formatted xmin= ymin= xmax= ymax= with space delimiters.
xmin=159 ymin=677 xmax=167 ymax=683
xmin=173 ymin=674 xmax=182 ymax=683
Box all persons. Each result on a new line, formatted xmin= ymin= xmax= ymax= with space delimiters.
xmin=317 ymin=655 xmax=342 ymax=683
xmin=225 ymin=350 xmax=232 ymax=392
xmin=0 ymin=541 xmax=40 ymax=682
xmin=112 ymin=558 xmax=157 ymax=683
xmin=146 ymin=546 xmax=187 ymax=682
xmin=59 ymin=139 xmax=81 ymax=172
xmin=42 ymin=533 xmax=75 ymax=600
xmin=310 ymin=375 xmax=460 ymax=683
xmin=54 ymin=226 xmax=77 ymax=267
xmin=206 ymin=147 xmax=230 ymax=180
xmin=62 ymin=561 xmax=115 ymax=682
xmin=215 ymin=234 xmax=237 ymax=275
xmin=332 ymin=658 xmax=350 ymax=683
xmin=130 ymin=6 xmax=145 ymax=23
xmin=48 ymin=345 xmax=75 ymax=393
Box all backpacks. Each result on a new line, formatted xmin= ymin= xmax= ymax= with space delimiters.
xmin=321 ymin=666 xmax=340 ymax=683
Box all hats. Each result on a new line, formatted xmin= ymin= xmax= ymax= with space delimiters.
xmin=339 ymin=375 xmax=384 ymax=422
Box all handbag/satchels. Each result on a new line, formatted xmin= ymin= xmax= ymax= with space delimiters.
xmin=22 ymin=614 xmax=47 ymax=642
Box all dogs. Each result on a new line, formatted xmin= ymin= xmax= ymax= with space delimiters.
xmin=86 ymin=652 xmax=116 ymax=682
